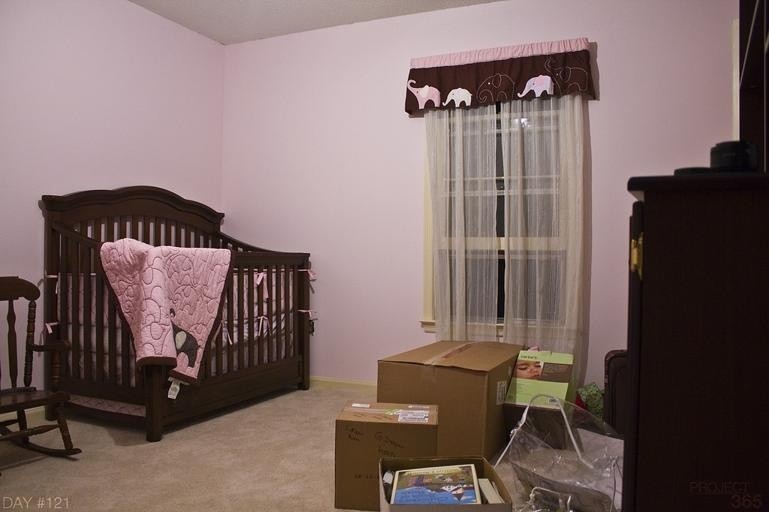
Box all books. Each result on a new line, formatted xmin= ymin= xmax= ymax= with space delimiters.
xmin=390 ymin=462 xmax=484 ymax=506
xmin=503 ymin=348 xmax=575 ymax=410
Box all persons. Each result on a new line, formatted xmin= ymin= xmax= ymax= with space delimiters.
xmin=512 ymin=360 xmax=544 ymax=378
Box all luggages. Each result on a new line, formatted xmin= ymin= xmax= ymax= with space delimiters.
xmin=601 ymin=347 xmax=627 ymax=435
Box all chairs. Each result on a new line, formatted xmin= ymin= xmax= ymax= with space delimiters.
xmin=0 ymin=276 xmax=82 ymax=455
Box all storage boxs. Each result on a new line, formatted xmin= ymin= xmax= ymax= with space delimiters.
xmin=335 ymin=340 xmax=579 ymax=512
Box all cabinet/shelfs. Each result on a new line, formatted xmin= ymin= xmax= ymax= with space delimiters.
xmin=622 ymin=0 xmax=769 ymax=512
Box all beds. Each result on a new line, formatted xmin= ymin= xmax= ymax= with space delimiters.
xmin=42 ymin=186 xmax=310 ymax=442
